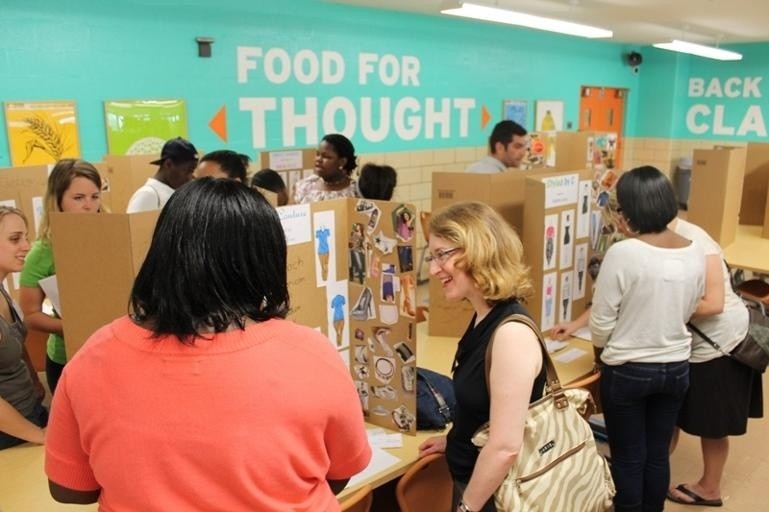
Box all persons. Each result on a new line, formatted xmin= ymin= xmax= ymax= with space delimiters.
xmin=576 ymin=247 xmax=585 ymax=291
xmin=546 ymin=278 xmax=552 ymax=318
xmin=0 ymin=205 xmax=50 ymax=453
xmin=18 ymin=158 xmax=110 ymax=400
xmin=561 ymin=277 xmax=570 ymax=321
xmin=583 ymin=184 xmax=588 ymax=214
xmin=316 ymin=204 xmax=415 ymax=347
xmin=43 ymin=174 xmax=373 ymax=511
xmin=125 ymin=132 xmax=397 ymax=215
xmin=424 ymin=202 xmax=546 ymax=512
xmin=460 ymin=119 xmax=527 ymax=175
xmin=547 ymin=226 xmax=555 ymax=267
xmin=563 ymin=214 xmax=570 ymax=245
xmin=587 ymin=165 xmax=706 ymax=512
xmin=548 ymin=189 xmax=763 ymax=507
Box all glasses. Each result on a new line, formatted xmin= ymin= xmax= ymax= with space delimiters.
xmin=423 ymin=247 xmax=464 ymax=264
xmin=616 ymin=208 xmax=623 ymax=214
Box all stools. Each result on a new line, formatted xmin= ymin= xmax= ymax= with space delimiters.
xmin=735 ymin=279 xmax=769 ymax=316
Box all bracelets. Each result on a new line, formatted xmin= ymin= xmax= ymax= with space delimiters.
xmin=457 ymin=497 xmax=469 ymax=512
xmin=374 ymin=358 xmax=394 ymax=380
xmin=391 ymin=405 xmax=414 ymax=432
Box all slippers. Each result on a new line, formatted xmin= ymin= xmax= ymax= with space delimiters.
xmin=667 ymin=480 xmax=724 ymax=508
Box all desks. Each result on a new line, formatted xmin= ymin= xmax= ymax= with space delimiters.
xmin=722 ymin=224 xmax=769 ymax=275
xmin=1 ymin=319 xmax=663 ymax=511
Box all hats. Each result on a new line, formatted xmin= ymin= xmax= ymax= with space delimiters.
xmin=149 ymin=136 xmax=199 ymax=165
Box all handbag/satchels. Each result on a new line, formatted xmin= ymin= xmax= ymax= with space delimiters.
xmin=731 ymin=308 xmax=769 ymax=371
xmin=416 ymin=366 xmax=456 ymax=431
xmin=465 ymin=386 xmax=618 ymax=512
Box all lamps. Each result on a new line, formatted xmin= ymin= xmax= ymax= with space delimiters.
xmin=440 ymin=3 xmax=613 ymax=40
xmin=652 ymin=35 xmax=742 ymax=61
